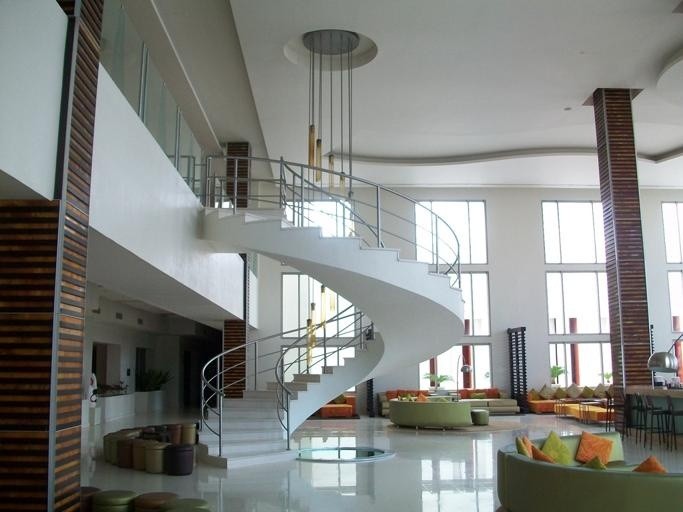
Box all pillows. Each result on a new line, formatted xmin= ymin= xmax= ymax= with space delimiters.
xmin=386 ymin=390 xmax=449 ymax=402
xmin=459 ymin=389 xmax=509 ymax=399
xmin=516 ymin=431 xmax=668 ymax=473
xmin=528 ymin=383 xmax=614 ymax=400
xmin=334 ymin=394 xmax=345 ymax=404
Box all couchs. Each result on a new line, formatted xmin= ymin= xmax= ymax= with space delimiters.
xmin=457 ymin=389 xmax=520 ymax=415
xmin=389 ymin=396 xmax=473 ymax=431
xmin=377 ymin=392 xmax=390 ymax=418
xmin=496 ymin=431 xmax=683 ymax=512
xmin=527 ymin=390 xmax=613 ymax=414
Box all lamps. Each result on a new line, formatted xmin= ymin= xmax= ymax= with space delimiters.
xmin=302 ymin=30 xmax=359 ymax=367
xmin=457 ymin=354 xmax=472 ymax=399
xmin=647 ymin=332 xmax=683 ymax=372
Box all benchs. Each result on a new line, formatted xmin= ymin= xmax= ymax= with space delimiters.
xmin=553 ymin=404 xmax=614 ymax=426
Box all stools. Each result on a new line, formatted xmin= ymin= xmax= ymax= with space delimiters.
xmin=81 ymin=423 xmax=210 ymax=511
xmin=471 ymin=409 xmax=489 ymax=425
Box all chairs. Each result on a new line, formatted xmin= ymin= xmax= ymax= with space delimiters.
xmin=319 ymin=396 xmax=356 ymax=419
xmin=605 ymin=390 xmax=683 ymax=452
